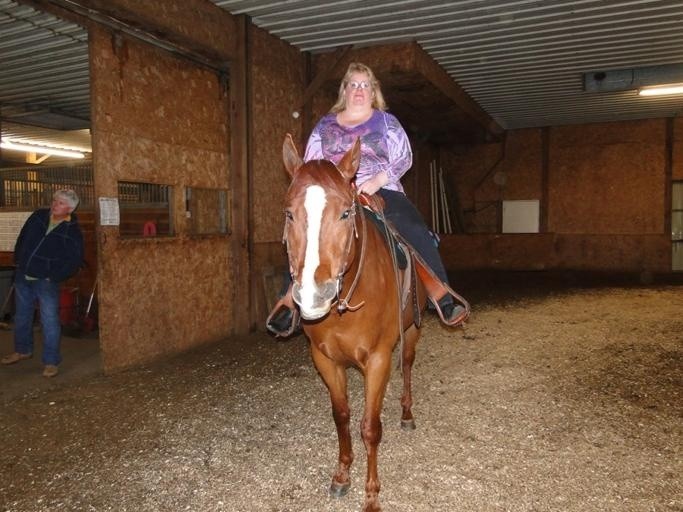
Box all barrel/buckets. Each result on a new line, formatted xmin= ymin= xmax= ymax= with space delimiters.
xmin=0 ymin=265 xmax=18 ymax=321
xmin=59 ymin=286 xmax=79 ymax=330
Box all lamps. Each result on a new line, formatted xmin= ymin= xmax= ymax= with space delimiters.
xmin=0 ymin=140 xmax=85 ymax=160
xmin=637 ymin=83 xmax=683 ymax=97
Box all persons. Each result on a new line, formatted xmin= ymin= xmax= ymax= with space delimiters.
xmin=263 ymin=61 xmax=467 ymax=332
xmin=0 ymin=189 xmax=82 ymax=378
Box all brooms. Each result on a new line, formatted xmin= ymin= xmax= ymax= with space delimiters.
xmin=0 ymin=279 xmax=16 ymax=330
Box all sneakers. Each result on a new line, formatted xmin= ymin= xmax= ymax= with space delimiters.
xmin=444 ymin=304 xmax=466 ymax=322
xmin=1 ymin=352 xmax=33 ymax=364
xmin=268 ymin=308 xmax=293 ymax=333
xmin=43 ymin=365 xmax=58 ymax=377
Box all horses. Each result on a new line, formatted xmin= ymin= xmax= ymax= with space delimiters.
xmin=277 ymin=131 xmax=430 ymax=512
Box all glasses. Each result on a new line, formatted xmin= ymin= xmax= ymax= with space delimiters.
xmin=348 ymin=81 xmax=371 ymax=89
